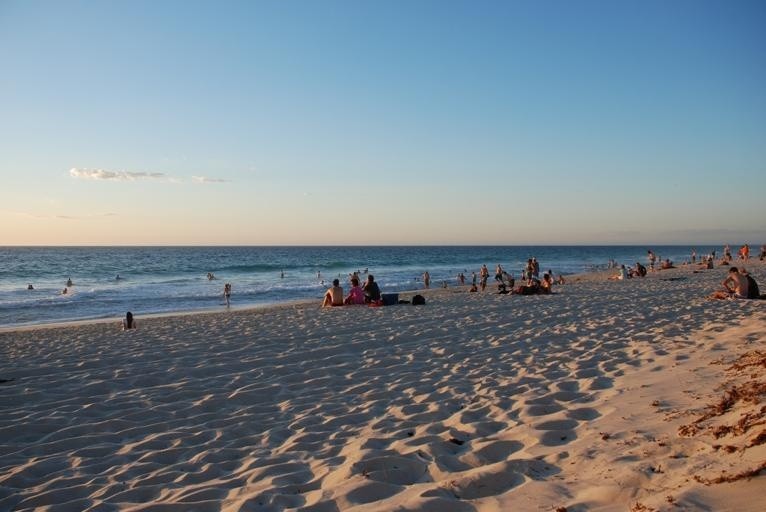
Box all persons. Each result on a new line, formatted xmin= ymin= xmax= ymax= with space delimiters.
xmin=422 ymin=272 xmax=430 ymax=288
xmin=28 ymin=285 xmax=30 ymax=289
xmin=692 ymin=243 xmax=766 ymax=299
xmin=121 ymin=311 xmax=137 ymax=331
xmin=608 ymin=251 xmax=673 ymax=280
xmin=457 ymin=257 xmax=564 ymax=294
xmin=30 ymin=285 xmax=33 ymax=289
xmin=62 ymin=287 xmax=67 ymax=295
xmin=321 ymin=268 xmax=380 ymax=308
xmin=224 ymin=283 xmax=232 ymax=309
xmin=66 ymin=277 xmax=73 ymax=288
